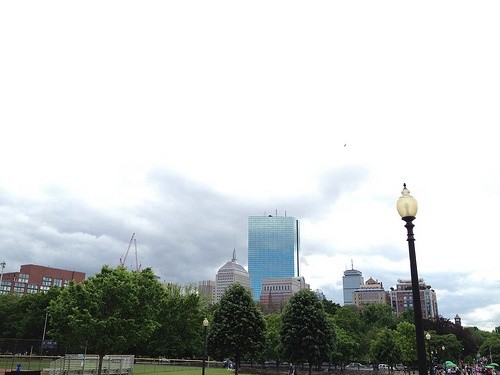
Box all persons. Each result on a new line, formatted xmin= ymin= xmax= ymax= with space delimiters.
xmin=433 ymin=356 xmax=500 ymax=375
xmin=379 ymin=366 xmax=406 ymax=375
xmin=288 ymin=362 xmax=296 ymax=375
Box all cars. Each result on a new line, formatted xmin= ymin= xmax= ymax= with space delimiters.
xmin=266 ymin=360 xmax=407 ymax=370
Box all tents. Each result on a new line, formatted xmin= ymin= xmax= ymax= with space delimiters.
xmin=444 ymin=361 xmax=457 ymax=369
xmin=483 ymin=363 xmax=500 ymax=372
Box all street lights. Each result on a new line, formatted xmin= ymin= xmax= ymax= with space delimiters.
xmin=202 ymin=318 xmax=209 ymax=374
xmin=425 ymin=332 xmax=452 ymax=375
xmin=397 ymin=183 xmax=428 ymax=375
